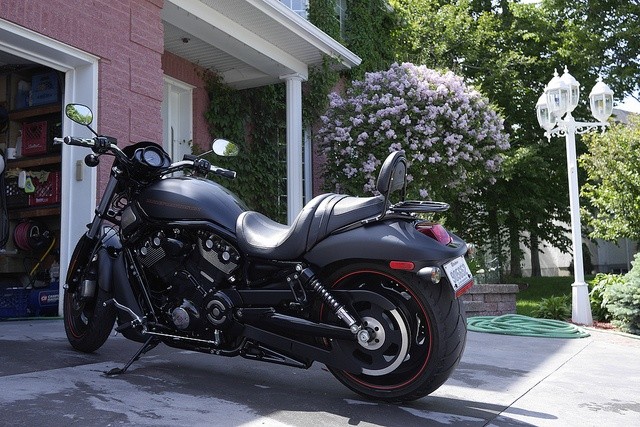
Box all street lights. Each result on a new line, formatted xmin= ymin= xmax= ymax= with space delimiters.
xmin=534 ymin=64 xmax=616 ymax=326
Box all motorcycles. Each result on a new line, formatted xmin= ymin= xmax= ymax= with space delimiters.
xmin=52 ymin=103 xmax=476 ymax=404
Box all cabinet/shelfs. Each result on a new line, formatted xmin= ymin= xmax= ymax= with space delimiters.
xmin=0 ymin=66 xmax=65 ymax=219
